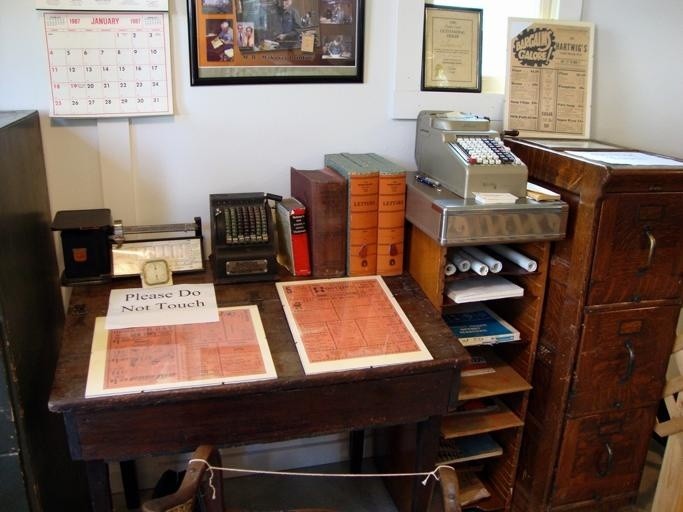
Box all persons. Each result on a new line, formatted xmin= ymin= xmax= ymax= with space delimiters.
xmin=325 ymin=0 xmax=345 ymax=25
xmin=238 ymin=23 xmax=256 ymax=49
xmin=212 ymin=20 xmax=233 ymax=48
xmin=326 ymin=35 xmax=344 ymax=57
xmin=268 ymin=0 xmax=302 ymax=48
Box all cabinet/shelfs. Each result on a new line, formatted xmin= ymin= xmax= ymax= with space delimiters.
xmin=368 ymin=225 xmax=552 ymax=512
xmin=0 ymin=110 xmax=106 ymax=512
xmin=508 ymin=139 xmax=682 ymax=512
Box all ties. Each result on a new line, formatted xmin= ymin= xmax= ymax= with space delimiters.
xmin=246 ymin=36 xmax=250 ymax=47
xmin=239 ymin=32 xmax=242 ymax=43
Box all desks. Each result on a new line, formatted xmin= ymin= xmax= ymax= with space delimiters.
xmin=47 ymin=264 xmax=471 ymax=512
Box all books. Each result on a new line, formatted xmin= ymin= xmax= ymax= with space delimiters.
xmin=274 ymin=152 xmax=310 ymax=278
xmin=443 ymin=306 xmax=522 ymax=347
xmin=526 ymin=181 xmax=561 ymax=202
xmin=448 ymin=277 xmax=524 ymax=303
xmin=290 ymin=164 xmax=348 ymax=278
xmin=354 ymin=153 xmax=407 ymax=276
xmin=325 ymin=152 xmax=378 ymax=278
xmin=429 ymin=441 xmax=504 ymax=467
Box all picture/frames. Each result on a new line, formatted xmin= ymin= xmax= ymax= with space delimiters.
xmin=186 ymin=1 xmax=365 ymax=86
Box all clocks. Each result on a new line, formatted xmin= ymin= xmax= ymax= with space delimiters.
xmin=139 ymin=257 xmax=174 ymax=289
